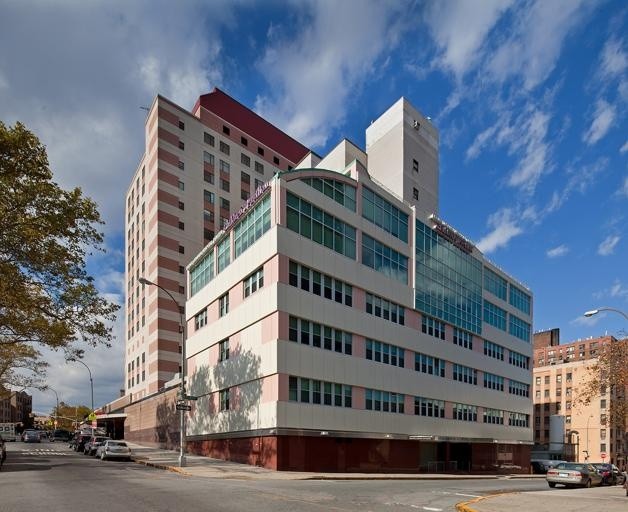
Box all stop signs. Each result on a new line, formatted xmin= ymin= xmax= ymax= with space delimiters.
xmin=601 ymin=453 xmax=606 ymax=458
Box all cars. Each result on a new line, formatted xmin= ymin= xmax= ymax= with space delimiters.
xmin=0 ymin=434 xmax=6 ymax=471
xmin=23 ymin=429 xmax=132 ymax=461
xmin=531 ymin=459 xmax=626 ymax=488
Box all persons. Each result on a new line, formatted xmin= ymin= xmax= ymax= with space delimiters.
xmin=0 ymin=440 xmax=6 ymax=471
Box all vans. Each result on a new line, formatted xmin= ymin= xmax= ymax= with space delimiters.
xmin=0 ymin=422 xmax=23 ymax=441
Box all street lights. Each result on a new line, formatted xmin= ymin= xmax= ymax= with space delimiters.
xmin=43 ymin=386 xmax=58 ymax=429
xmin=138 ymin=277 xmax=186 ymax=467
xmin=586 ymin=415 xmax=594 ymax=462
xmin=66 ymin=358 xmax=93 ymax=437
xmin=584 ymin=306 xmax=628 ymax=320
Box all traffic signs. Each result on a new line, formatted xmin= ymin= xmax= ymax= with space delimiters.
xmin=175 ymin=396 xmax=198 ymax=411
